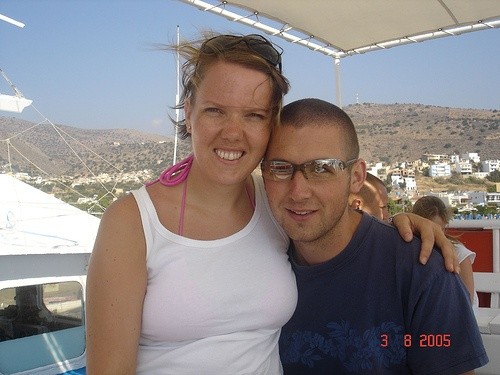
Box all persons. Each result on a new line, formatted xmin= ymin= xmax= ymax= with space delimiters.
xmin=348 ymin=168 xmax=390 ymax=226
xmin=84 ymin=30 xmax=459 ymax=375
xmin=259 ymin=96 xmax=490 ymax=375
xmin=412 ymin=194 xmax=479 ymax=318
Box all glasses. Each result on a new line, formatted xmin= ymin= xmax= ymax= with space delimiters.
xmin=193 ymin=34 xmax=283 ymax=77
xmin=261 ymin=157 xmax=359 ymax=182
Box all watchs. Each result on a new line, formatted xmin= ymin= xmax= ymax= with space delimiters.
xmin=388 ymin=211 xmax=400 ymax=226
